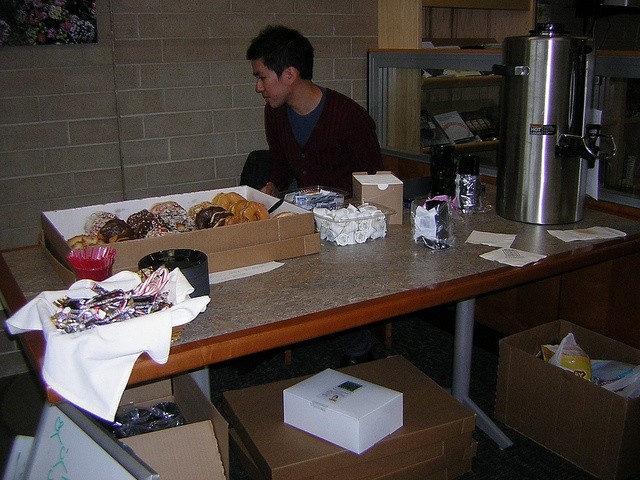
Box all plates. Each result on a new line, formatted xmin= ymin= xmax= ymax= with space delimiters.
xmin=138 ymin=248 xmax=212 ymax=297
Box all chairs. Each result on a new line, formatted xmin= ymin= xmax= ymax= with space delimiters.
xmin=239 ymin=149 xmax=395 ymax=365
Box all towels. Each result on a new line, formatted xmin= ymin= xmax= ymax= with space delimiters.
xmin=6 ymin=267 xmax=211 ymax=423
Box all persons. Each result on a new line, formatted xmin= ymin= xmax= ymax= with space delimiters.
xmin=245 ymin=23 xmax=383 ymax=195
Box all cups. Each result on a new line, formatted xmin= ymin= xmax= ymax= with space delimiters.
xmin=65 ymin=244 xmax=117 ymax=281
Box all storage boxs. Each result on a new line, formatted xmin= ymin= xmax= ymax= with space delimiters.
xmin=283 ymin=367 xmax=403 ymax=455
xmin=220 ymin=352 xmax=480 ymax=479
xmin=492 ymin=317 xmax=640 ymax=479
xmin=350 ymin=169 xmax=404 ymax=225
xmin=92 ymin=373 xmax=230 ymax=479
xmin=40 ymin=184 xmax=322 ymax=279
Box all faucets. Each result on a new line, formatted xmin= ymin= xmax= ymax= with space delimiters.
xmin=555 ymin=124 xmax=602 ymax=169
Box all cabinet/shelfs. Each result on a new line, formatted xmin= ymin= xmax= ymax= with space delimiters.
xmin=588 ymin=47 xmax=640 ymax=208
xmin=377 ymin=0 xmax=537 ymax=155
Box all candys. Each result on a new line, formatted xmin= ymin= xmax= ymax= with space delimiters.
xmin=50 ymin=279 xmax=172 ymax=332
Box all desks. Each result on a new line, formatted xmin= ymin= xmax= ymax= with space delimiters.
xmin=1 ymin=182 xmax=640 ymax=452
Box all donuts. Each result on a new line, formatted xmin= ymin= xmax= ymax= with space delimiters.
xmin=65 ymin=211 xmax=136 ymax=248
xmin=128 ymin=201 xmax=195 ymax=239
xmin=190 ymin=192 xmax=270 ymax=229
